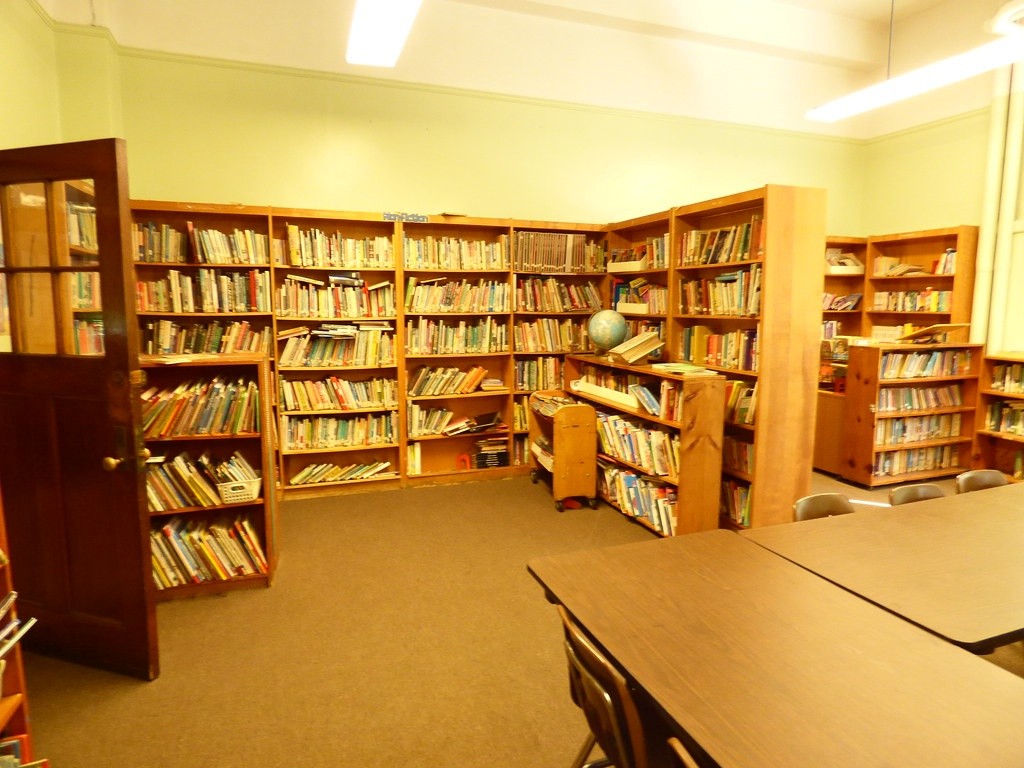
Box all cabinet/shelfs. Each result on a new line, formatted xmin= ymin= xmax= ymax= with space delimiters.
xmin=62 ymin=182 xmax=1024 ymax=600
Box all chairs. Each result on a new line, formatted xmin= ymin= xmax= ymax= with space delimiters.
xmin=792 ymin=491 xmax=856 ymax=523
xmin=955 ymin=468 xmax=1009 ymax=493
xmin=887 ymin=483 xmax=945 ymax=508
xmin=550 ymin=603 xmax=686 ymax=768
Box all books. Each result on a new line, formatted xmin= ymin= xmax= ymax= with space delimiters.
xmin=984 ymin=363 xmax=1024 ymax=477
xmin=0 ymin=550 xmax=52 ymax=768
xmin=268 ymin=223 xmax=396 ymax=485
xmin=818 ymin=247 xmax=864 ymax=381
xmin=515 ymin=227 xmax=606 ymax=463
xmin=530 ymin=329 xmax=720 ymax=539
xmin=876 ymin=351 xmax=972 ymax=475
xmin=401 ymin=209 xmax=512 ymax=479
xmin=64 ymin=209 xmax=269 ymax=590
xmin=871 ymin=249 xmax=955 ymax=342
xmin=607 ymin=216 xmax=762 ymax=526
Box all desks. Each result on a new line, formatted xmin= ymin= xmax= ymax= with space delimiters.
xmin=739 ymin=478 xmax=1024 ymax=653
xmin=525 ymin=529 xmax=1024 ymax=767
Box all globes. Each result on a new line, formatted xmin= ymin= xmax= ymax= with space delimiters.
xmin=586 ymin=309 xmax=628 ymax=357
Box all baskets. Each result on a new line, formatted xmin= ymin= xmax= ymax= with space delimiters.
xmin=215 ymin=469 xmax=262 ymax=504
